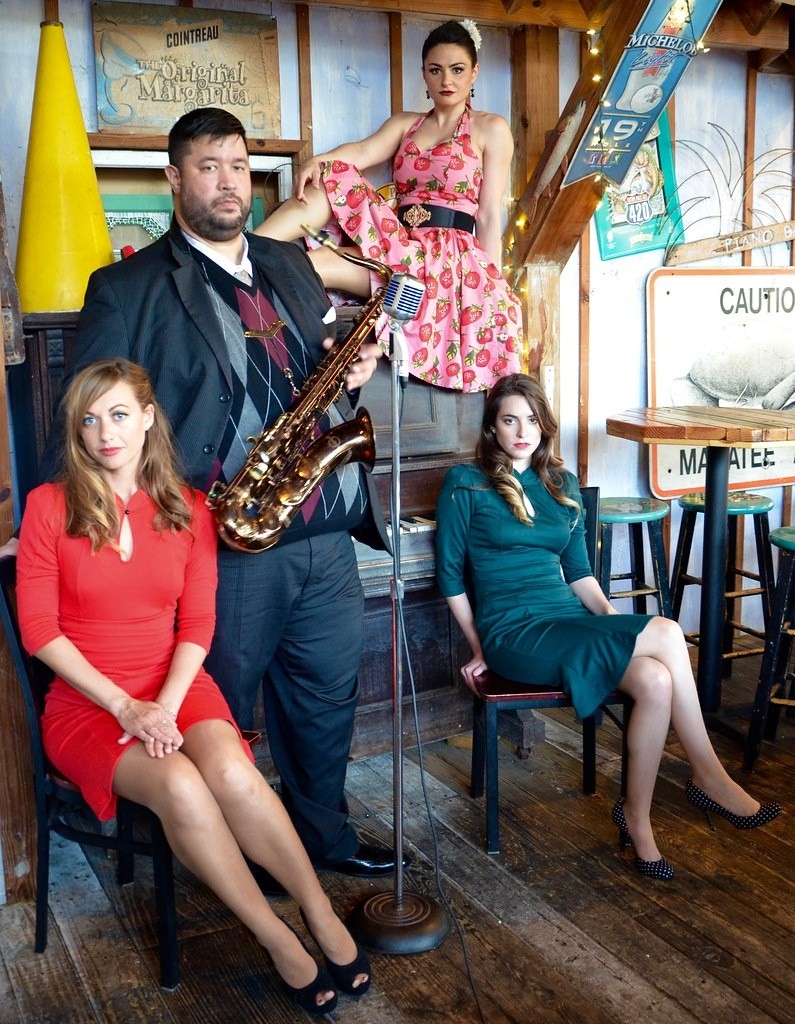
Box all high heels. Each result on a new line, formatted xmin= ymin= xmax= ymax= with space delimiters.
xmin=611 ymin=797 xmax=673 ymax=882
xmin=686 ymin=779 xmax=782 ymax=834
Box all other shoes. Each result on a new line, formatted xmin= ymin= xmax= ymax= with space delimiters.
xmin=259 ymin=919 xmax=340 ymax=1015
xmin=300 ymin=903 xmax=370 ymax=997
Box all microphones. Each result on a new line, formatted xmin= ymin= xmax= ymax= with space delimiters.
xmin=381 ymin=273 xmax=425 ymax=389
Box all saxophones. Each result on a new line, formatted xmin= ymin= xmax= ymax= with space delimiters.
xmin=202 ymin=216 xmax=398 ymax=558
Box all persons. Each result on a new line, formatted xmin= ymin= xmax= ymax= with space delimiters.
xmin=434 ymin=372 xmax=782 ymax=878
xmin=252 ymin=19 xmax=522 ymax=392
xmin=76 ymin=108 xmax=418 ymax=895
xmin=17 ymin=359 xmax=376 ymax=1013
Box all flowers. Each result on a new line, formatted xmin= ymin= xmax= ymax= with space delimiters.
xmin=459 ymin=17 xmax=482 ymax=53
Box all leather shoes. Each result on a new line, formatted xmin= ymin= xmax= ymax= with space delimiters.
xmin=242 ymin=850 xmax=292 ymax=901
xmin=321 ymin=843 xmax=410 ymax=878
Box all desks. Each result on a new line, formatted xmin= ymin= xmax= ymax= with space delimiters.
xmin=604 ymin=407 xmax=794 ymax=744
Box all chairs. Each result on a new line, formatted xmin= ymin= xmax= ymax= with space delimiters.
xmin=0 ymin=553 xmax=260 ymax=992
xmin=468 ymin=487 xmax=632 ymax=856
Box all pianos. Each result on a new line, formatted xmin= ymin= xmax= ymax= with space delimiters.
xmin=1 ymin=298 xmax=550 ymax=760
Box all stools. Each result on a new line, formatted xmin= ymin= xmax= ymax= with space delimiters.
xmin=669 ymin=492 xmax=786 ymax=705
xmin=583 ymin=497 xmax=673 ymax=726
xmin=744 ymin=526 xmax=795 ymax=774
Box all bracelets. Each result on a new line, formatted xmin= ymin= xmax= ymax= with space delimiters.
xmin=161 ymin=707 xmax=178 ymax=718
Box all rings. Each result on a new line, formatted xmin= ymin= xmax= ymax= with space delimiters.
xmin=161 ymin=719 xmax=166 ymax=724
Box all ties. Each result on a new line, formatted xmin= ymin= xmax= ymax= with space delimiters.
xmin=234 ymin=269 xmax=252 ymax=288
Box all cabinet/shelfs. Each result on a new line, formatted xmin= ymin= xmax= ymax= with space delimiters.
xmin=21 ymin=306 xmax=495 ymax=785
